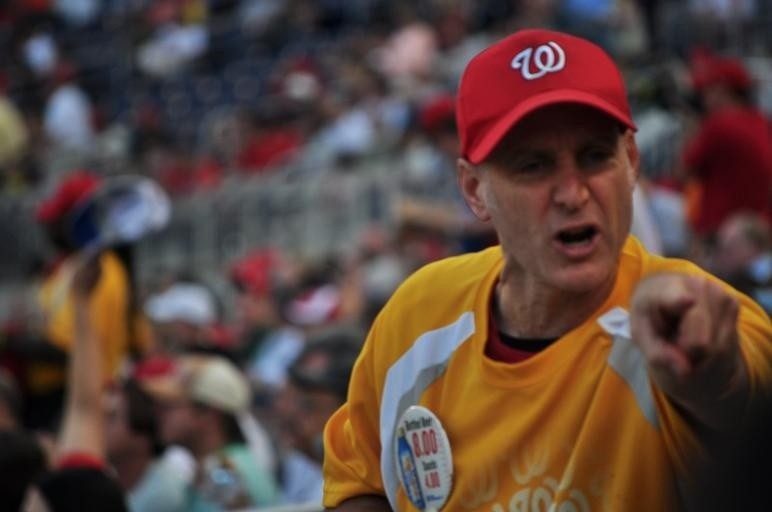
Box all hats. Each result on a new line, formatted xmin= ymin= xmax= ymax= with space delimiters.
xmin=77 ymin=352 xmax=252 ymax=410
xmin=143 ymin=282 xmax=218 ymax=328
xmin=456 ymin=27 xmax=641 ymax=167
